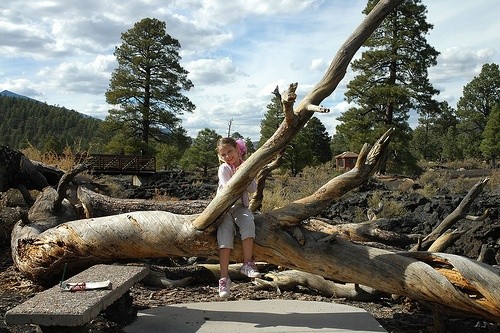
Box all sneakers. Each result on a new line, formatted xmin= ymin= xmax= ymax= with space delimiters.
xmin=239 ymin=260 xmax=261 ymax=277
xmin=219 ymin=275 xmax=231 ymax=297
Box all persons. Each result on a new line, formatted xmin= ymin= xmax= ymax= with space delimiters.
xmin=0 ymin=145 xmax=50 ymax=210
xmin=215 ymin=136 xmax=262 ymax=297
